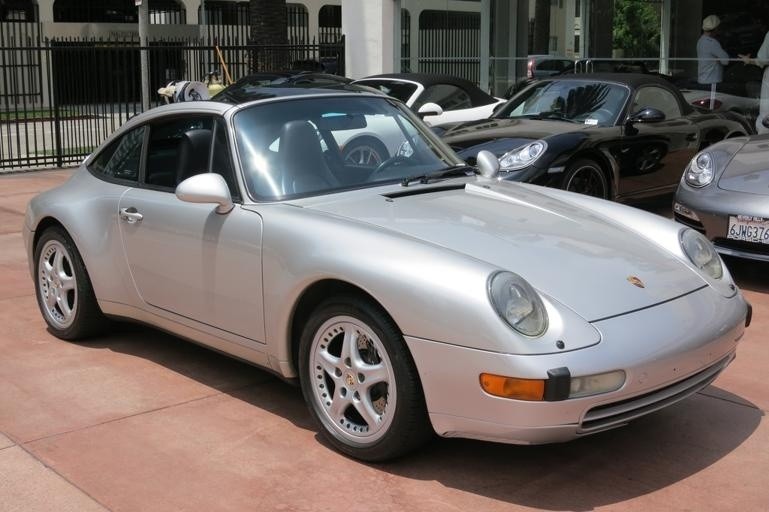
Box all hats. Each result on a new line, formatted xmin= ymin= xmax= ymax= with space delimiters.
xmin=702 ymin=15 xmax=720 ymax=31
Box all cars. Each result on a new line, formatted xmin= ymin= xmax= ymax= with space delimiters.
xmin=526 ymin=55 xmax=577 ymax=79
xmin=209 ymin=70 xmax=355 ymax=106
xmin=390 ymin=72 xmax=756 ymax=204
xmin=673 ymin=133 xmax=768 ymax=272
xmin=23 ymin=81 xmax=754 ymax=464
xmin=268 ymin=73 xmax=510 ymax=171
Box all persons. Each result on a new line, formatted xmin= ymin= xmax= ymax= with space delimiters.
xmin=736 ymin=31 xmax=769 ymax=101
xmin=694 ymin=14 xmax=729 ymax=90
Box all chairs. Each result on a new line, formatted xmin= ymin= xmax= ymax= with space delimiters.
xmin=176 ymin=129 xmax=239 ymax=200
xmin=277 ymin=120 xmax=340 ymax=196
